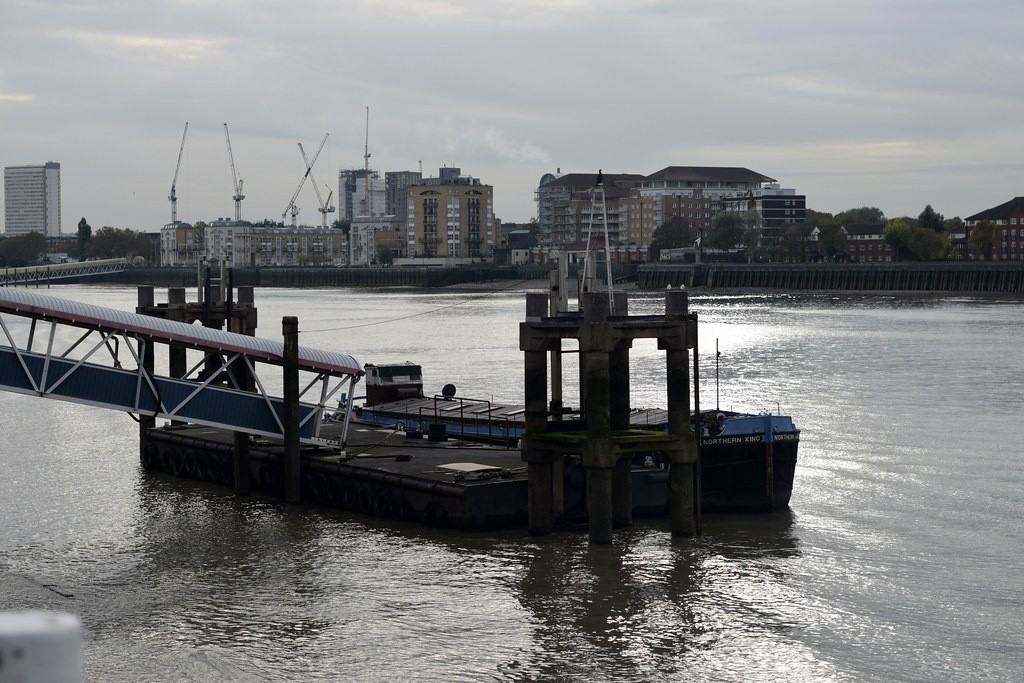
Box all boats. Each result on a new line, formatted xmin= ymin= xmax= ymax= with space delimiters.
xmin=339 ymin=359 xmax=798 ymax=520
